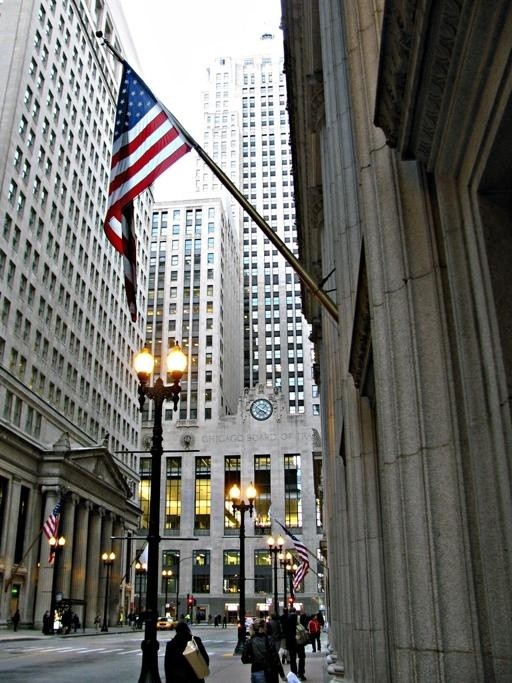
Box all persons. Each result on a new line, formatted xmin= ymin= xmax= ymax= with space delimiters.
xmin=164 ymin=621 xmax=209 ymax=682
xmin=12 ymin=607 xmax=21 ymax=631
xmin=214 ymin=614 xmax=219 ymax=628
xmin=207 ymin=613 xmax=212 ymax=624
xmin=268 ymin=606 xmax=324 ymax=681
xmin=217 ymin=612 xmax=222 ymax=623
xmin=221 ymin=614 xmax=227 ymax=629
xmin=93 ymin=615 xmax=101 ymax=630
xmin=42 ymin=609 xmax=50 ymax=634
xmin=54 ymin=607 xmax=80 ymax=633
xmin=240 ymin=617 xmax=289 ymax=683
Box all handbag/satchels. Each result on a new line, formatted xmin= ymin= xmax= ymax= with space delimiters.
xmin=181 ymin=634 xmax=210 ymax=679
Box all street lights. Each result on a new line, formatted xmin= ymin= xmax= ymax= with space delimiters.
xmin=162 ymin=570 xmax=173 ymax=616
xmin=49 ymin=536 xmax=66 ymax=633
xmin=229 ymin=480 xmax=256 ymax=656
xmin=267 ymin=535 xmax=297 ymax=612
xmin=134 ymin=340 xmax=188 ymax=683
xmin=136 ymin=562 xmax=147 ymax=612
xmin=101 ymin=552 xmax=115 ymax=631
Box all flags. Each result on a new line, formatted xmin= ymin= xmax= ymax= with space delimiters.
xmin=42 ymin=494 xmax=69 ymax=566
xmin=93 ymin=25 xmax=195 ymax=322
xmin=138 ymin=542 xmax=148 ymax=574
xmin=282 ymin=541 xmax=302 ymax=567
xmin=267 ymin=511 xmax=313 ymax=591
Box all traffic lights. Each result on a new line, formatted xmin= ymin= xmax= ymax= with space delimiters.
xmin=188 ymin=596 xmax=196 ymax=606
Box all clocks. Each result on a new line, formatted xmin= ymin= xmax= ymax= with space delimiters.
xmin=250 ymin=398 xmax=274 ymax=422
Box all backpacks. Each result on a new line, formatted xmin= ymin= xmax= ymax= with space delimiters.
xmin=294 ymin=614 xmax=309 ymax=644
xmin=263 ymin=637 xmax=278 ymax=683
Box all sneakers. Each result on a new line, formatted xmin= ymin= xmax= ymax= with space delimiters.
xmin=296 ymin=673 xmax=306 ymax=680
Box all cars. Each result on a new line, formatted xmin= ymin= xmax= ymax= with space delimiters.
xmin=157 ymin=617 xmax=178 ymax=629
